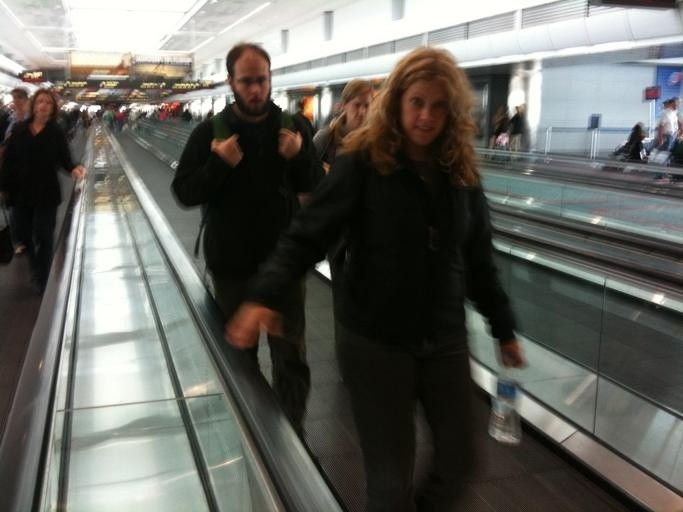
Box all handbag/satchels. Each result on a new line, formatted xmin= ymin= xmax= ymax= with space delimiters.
xmin=0 ymin=225 xmax=14 ymax=266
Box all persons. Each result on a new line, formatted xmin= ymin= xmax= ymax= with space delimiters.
xmin=489 ymin=102 xmax=524 ymax=153
xmin=222 ymin=47 xmax=528 ymax=508
xmin=312 ymin=79 xmax=375 ymax=170
xmin=81 ymin=105 xmax=192 ymax=133
xmin=1 ymin=87 xmax=87 ymax=295
xmin=168 ymin=43 xmax=317 ymax=462
xmin=289 ymin=101 xmax=314 ymax=139
xmin=610 ymin=97 xmax=683 ymax=167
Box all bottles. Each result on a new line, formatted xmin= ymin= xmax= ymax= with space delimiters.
xmin=489 ymin=361 xmax=524 ymax=446
